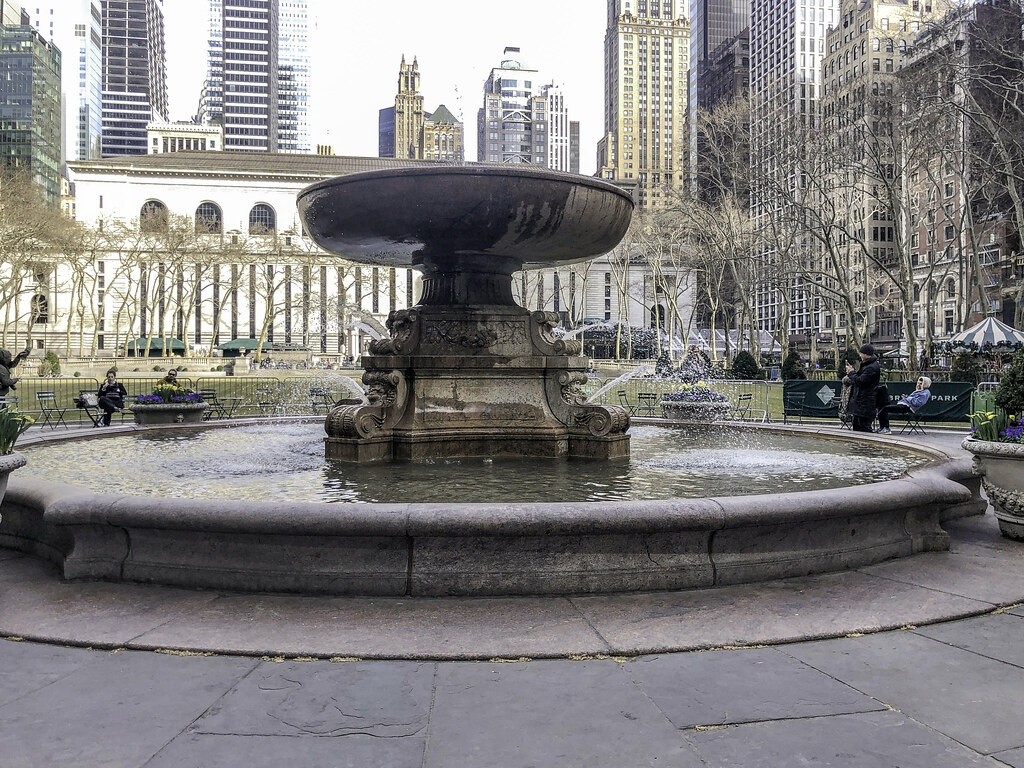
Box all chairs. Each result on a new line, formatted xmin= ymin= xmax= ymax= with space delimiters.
xmin=782 ymin=392 xmax=806 ymax=425
xmin=831 ymin=397 xmax=853 ymax=430
xmin=310 ymin=387 xmax=336 ymax=415
xmin=37 ymin=391 xmax=69 ymax=430
xmin=200 ymin=389 xmax=229 ymax=419
xmin=257 ymin=389 xmax=279 ymax=417
xmin=637 ymin=392 xmax=657 ymax=417
xmin=900 ymin=410 xmax=925 ymax=436
xmin=120 ymin=395 xmax=137 ymax=425
xmin=0 ymin=396 xmax=20 ymax=406
xmin=730 ymin=393 xmax=753 ymax=421
xmin=617 ymin=390 xmax=637 ymax=417
xmin=79 ymin=390 xmax=106 ymax=427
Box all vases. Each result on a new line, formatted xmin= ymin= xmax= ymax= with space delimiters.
xmin=961 ymin=435 xmax=1024 ymax=543
xmin=661 ymin=402 xmax=730 ymax=420
xmin=128 ymin=403 xmax=208 ymax=423
xmin=0 ymin=452 xmax=25 ymax=522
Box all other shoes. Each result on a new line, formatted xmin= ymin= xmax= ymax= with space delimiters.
xmin=875 ymin=427 xmax=892 ymax=434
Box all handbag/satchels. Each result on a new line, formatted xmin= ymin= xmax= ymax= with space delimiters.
xmin=877 ymin=385 xmax=891 ymax=408
xmin=106 ymin=393 xmax=120 ymax=398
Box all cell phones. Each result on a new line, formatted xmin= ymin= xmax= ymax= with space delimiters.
xmin=845 ymin=359 xmax=848 ymax=366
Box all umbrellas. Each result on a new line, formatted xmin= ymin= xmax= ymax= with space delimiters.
xmin=948 ymin=317 xmax=1024 ymax=352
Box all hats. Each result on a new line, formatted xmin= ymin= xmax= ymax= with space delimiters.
xmin=859 ymin=344 xmax=873 ymax=356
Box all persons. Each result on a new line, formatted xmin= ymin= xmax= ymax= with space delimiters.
xmin=0 ymin=349 xmax=19 ymax=408
xmin=878 ymin=376 xmax=932 ymax=435
xmin=163 ymin=369 xmax=180 ymax=388
xmin=845 ymin=343 xmax=880 ymax=432
xmin=97 ymin=371 xmax=127 ymax=427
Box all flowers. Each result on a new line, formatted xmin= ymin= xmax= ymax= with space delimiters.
xmin=969 ymin=411 xmax=1024 ymax=441
xmin=0 ymin=405 xmax=34 ymax=456
xmin=663 ymin=386 xmax=726 ymax=402
xmin=135 ymin=384 xmax=204 ymax=403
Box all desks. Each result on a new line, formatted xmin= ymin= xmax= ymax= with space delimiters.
xmin=217 ymin=397 xmax=244 ymax=420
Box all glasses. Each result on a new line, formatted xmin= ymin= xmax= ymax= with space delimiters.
xmin=169 ymin=373 xmax=175 ymax=376
